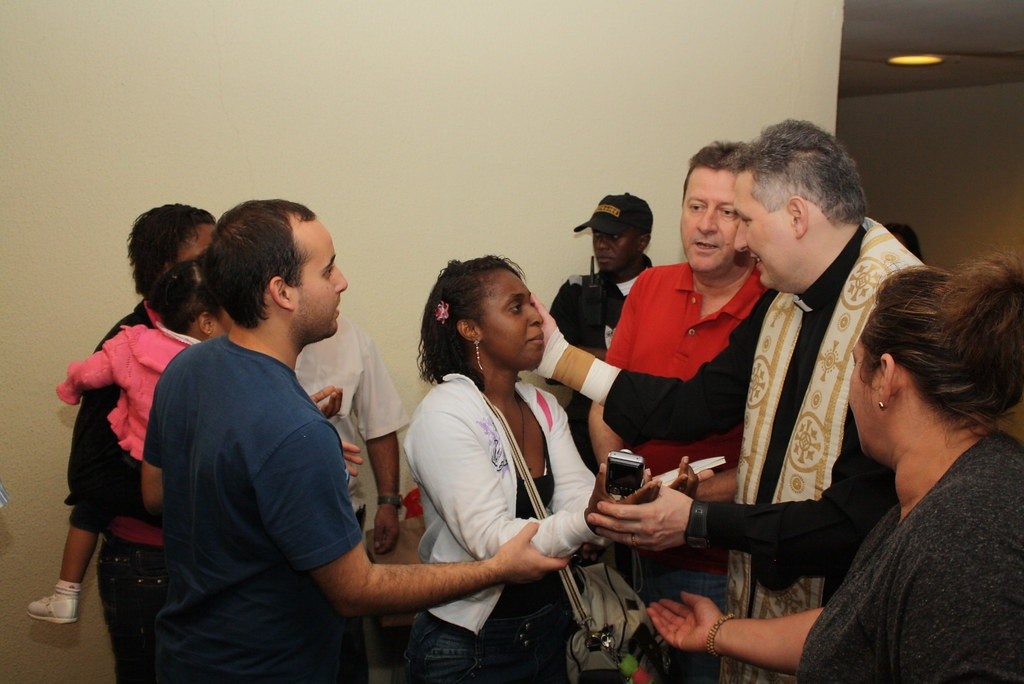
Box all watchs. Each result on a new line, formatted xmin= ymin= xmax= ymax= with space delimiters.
xmin=683 ymin=499 xmax=710 ymax=549
xmin=377 ymin=495 xmax=402 ymax=509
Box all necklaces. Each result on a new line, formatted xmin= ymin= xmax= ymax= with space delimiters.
xmin=514 ymin=396 xmax=525 ymax=457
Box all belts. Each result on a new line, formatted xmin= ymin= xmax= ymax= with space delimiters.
xmin=100 ymin=538 xmax=166 ymax=566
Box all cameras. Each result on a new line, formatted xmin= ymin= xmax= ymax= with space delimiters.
xmin=605 ymin=449 xmax=646 ymax=502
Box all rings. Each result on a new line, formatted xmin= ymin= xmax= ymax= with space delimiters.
xmin=632 ymin=536 xmax=636 ymax=546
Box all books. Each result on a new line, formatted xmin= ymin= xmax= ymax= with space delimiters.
xmin=652 ymin=456 xmax=726 ymax=486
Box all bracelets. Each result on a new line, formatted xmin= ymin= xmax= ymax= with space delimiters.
xmin=706 ymin=614 xmax=735 ymax=657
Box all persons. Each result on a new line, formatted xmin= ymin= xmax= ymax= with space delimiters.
xmin=293 ymin=317 xmax=412 ymax=684
xmin=885 ymin=221 xmax=923 ymax=260
xmin=402 ymin=255 xmax=698 ymax=683
xmin=528 ymin=118 xmax=928 ymax=684
xmin=587 ymin=140 xmax=772 ymax=684
xmin=647 ymin=256 xmax=1024 ymax=684
xmin=26 ymin=203 xmax=226 ymax=684
xmin=542 ymin=193 xmax=655 ymax=477
xmin=138 ymin=200 xmax=572 ymax=684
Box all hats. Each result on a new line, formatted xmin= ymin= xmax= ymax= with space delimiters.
xmin=574 ymin=192 xmax=654 ymax=236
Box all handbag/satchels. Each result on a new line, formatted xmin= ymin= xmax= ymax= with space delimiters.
xmin=566 ymin=563 xmax=674 ymax=684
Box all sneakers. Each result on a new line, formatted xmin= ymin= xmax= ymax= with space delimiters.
xmin=26 ymin=595 xmax=79 ymax=624
